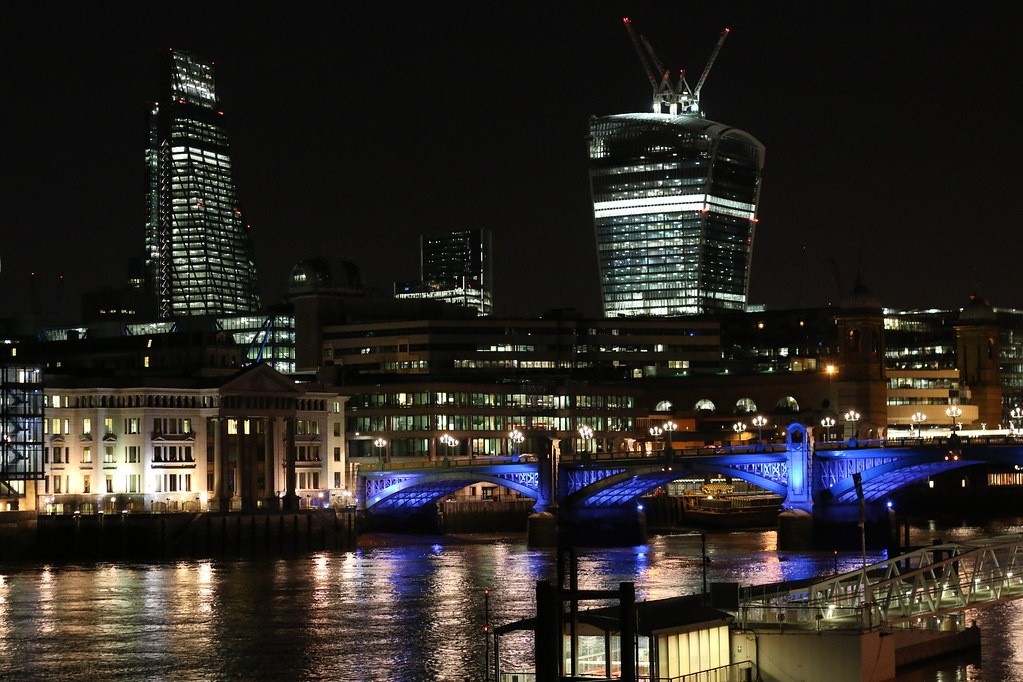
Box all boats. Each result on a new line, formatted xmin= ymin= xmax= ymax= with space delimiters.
xmin=683 ymin=504 xmax=782 ymax=531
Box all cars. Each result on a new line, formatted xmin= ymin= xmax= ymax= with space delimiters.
xmin=518 ymin=453 xmax=538 ymax=463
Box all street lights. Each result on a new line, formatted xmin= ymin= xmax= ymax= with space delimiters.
xmin=944 ymin=406 xmax=964 ymax=440
xmin=1010 ymin=407 xmax=1023 ymax=437
xmin=751 ymin=414 xmax=767 ymax=453
xmin=663 ymin=421 xmax=677 ymax=462
xmin=580 ymin=426 xmax=594 ymax=463
xmin=649 ymin=426 xmax=662 ymax=451
xmin=439 ymin=434 xmax=459 ymax=468
xmin=844 ymin=410 xmax=861 ymax=447
xmin=509 ymin=430 xmax=525 ymax=462
xmin=912 ymin=412 xmax=927 ymax=443
xmin=374 ymin=438 xmax=386 ymax=470
xmin=732 ymin=422 xmax=747 ymax=446
xmin=821 ymin=417 xmax=836 ymax=442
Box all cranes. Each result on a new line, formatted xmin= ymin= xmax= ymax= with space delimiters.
xmin=619 ymin=14 xmax=731 ymax=115
